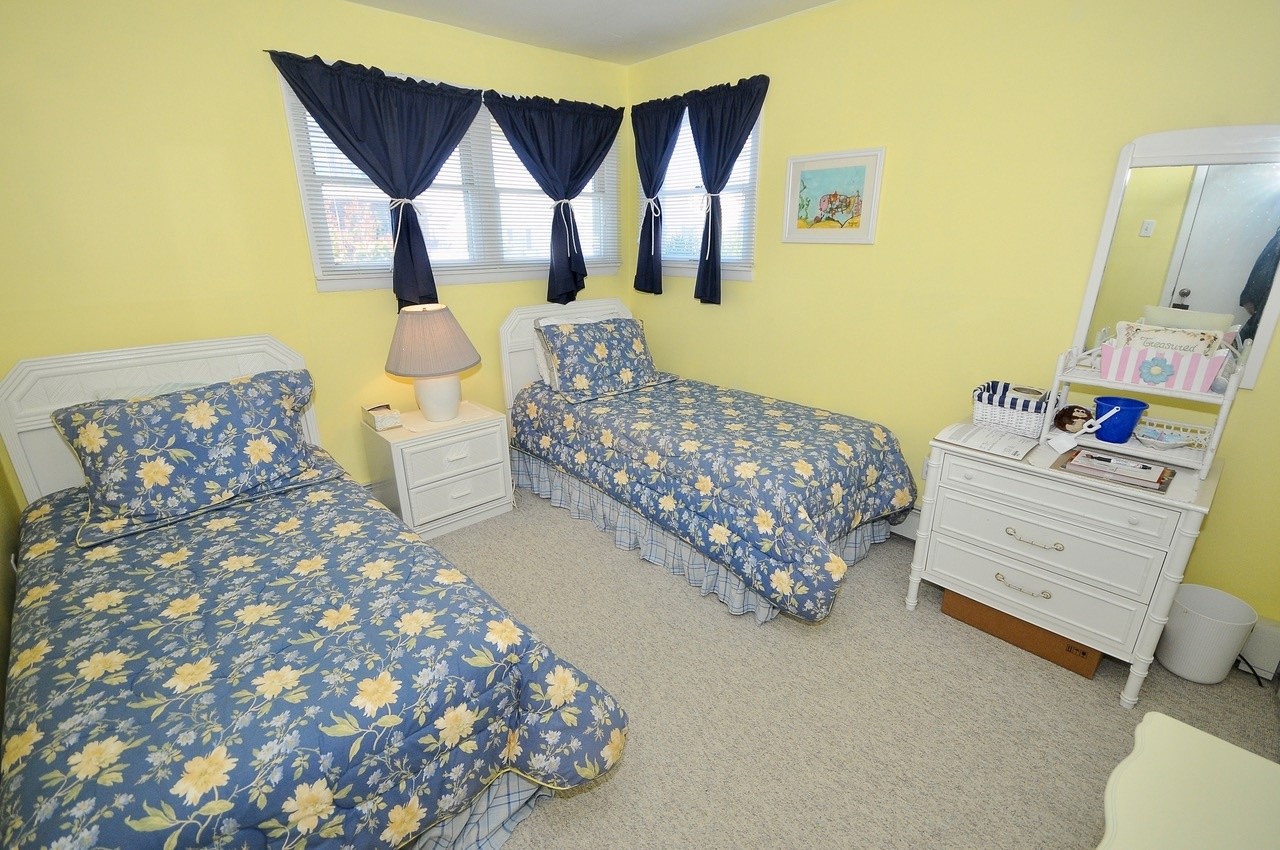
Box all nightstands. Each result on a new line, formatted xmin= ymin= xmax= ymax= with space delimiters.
xmin=361 ymin=401 xmax=514 ymax=541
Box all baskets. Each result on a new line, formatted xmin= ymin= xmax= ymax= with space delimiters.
xmin=973 ymin=381 xmax=1061 ymax=439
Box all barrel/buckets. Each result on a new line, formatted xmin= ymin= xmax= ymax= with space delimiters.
xmin=1154 ymin=583 xmax=1258 ymax=685
xmin=1094 ymin=396 xmax=1149 ymax=444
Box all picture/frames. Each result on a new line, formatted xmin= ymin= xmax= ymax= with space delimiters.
xmin=781 ymin=144 xmax=885 ymax=244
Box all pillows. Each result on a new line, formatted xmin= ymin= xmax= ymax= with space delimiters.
xmin=533 ymin=308 xmax=620 ymax=382
xmin=50 ymin=368 xmax=345 ymax=546
xmin=534 ymin=318 xmax=678 ymax=403
xmin=535 ymin=312 xmax=623 ymax=390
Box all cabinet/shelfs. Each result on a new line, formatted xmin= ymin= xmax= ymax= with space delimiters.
xmin=906 ymin=413 xmax=1220 ymax=711
xmin=1040 ymin=346 xmax=1251 ymax=482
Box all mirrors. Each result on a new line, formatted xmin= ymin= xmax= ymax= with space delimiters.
xmin=1074 ymin=124 xmax=1279 ymax=390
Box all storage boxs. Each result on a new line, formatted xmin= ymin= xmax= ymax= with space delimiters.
xmin=1134 ymin=305 xmax=1242 ymax=345
xmin=1101 ymin=321 xmax=1228 ymax=392
xmin=941 ymin=587 xmax=1102 ymax=679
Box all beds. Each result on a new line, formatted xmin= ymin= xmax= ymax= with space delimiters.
xmin=0 ymin=336 xmax=625 ymax=850
xmin=499 ymin=297 xmax=919 ymax=621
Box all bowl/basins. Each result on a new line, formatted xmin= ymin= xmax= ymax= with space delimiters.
xmin=1133 ymin=425 xmax=1195 ymax=451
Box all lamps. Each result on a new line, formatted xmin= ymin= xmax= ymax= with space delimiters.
xmin=385 ymin=303 xmax=482 ymax=423
